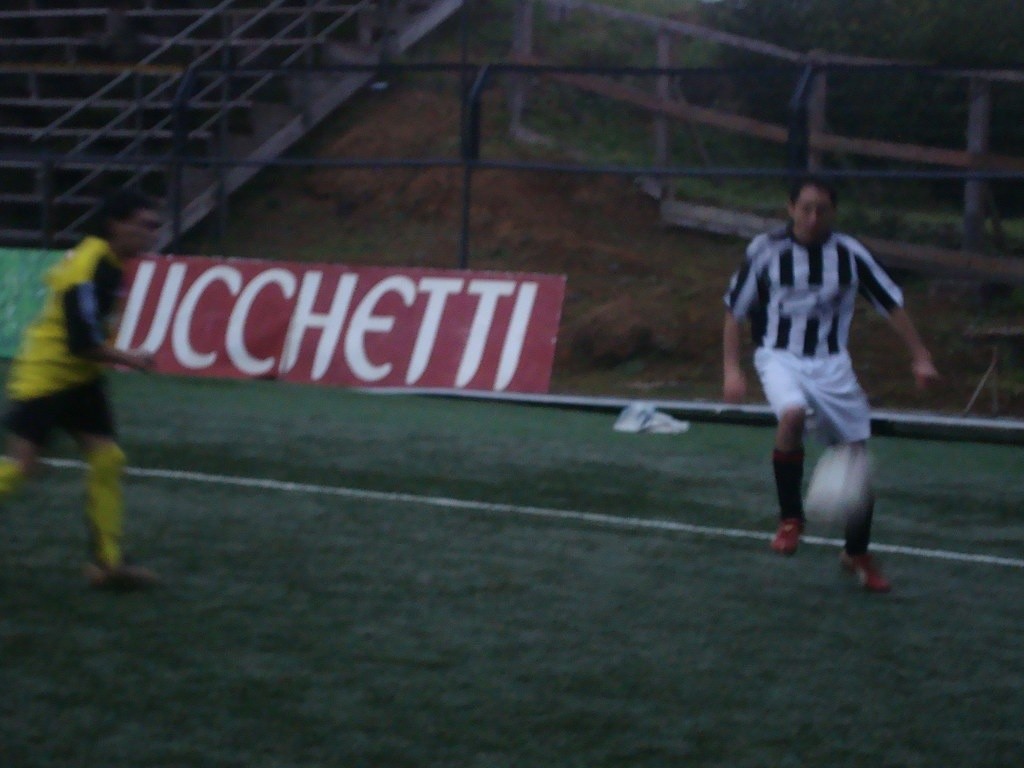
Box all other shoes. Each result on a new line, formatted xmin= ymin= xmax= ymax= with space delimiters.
xmin=88 ymin=564 xmax=159 ymax=590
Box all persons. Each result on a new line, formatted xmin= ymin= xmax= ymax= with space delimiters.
xmin=0 ymin=197 xmax=165 ymax=590
xmin=724 ymin=177 xmax=938 ymax=594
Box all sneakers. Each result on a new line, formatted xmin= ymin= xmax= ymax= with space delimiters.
xmin=772 ymin=518 xmax=803 ymax=554
xmin=837 ymin=550 xmax=889 ymax=593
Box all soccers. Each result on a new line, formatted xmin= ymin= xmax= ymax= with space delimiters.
xmin=799 ymin=455 xmax=877 ymax=527
